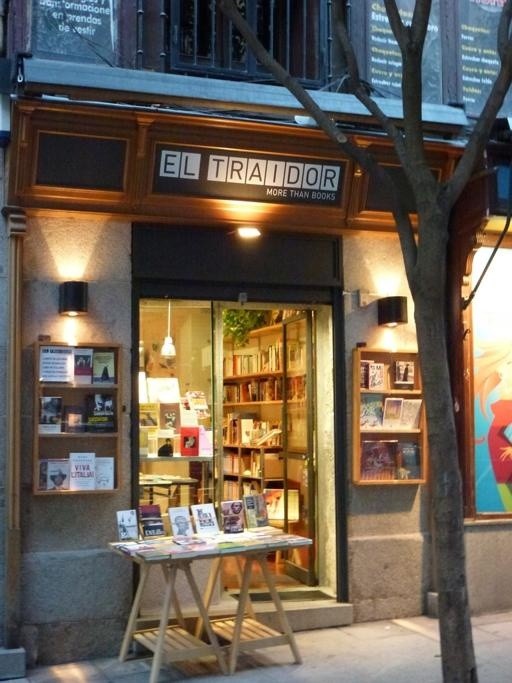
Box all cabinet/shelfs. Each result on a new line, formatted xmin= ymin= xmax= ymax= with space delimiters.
xmin=31 ymin=342 xmax=126 ymax=499
xmin=352 ymin=346 xmax=429 ymax=489
xmin=205 ymin=311 xmax=317 ymax=568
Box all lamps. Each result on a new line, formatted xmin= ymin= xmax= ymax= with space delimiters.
xmin=58 ymin=281 xmax=89 ymax=317
xmin=159 ymin=299 xmax=177 ymax=359
xmin=377 ymin=296 xmax=408 ymax=328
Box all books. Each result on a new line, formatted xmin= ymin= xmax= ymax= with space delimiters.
xmin=139 ymin=372 xmax=214 ymax=512
xmin=34 ymin=344 xmax=106 ymax=495
xmin=358 ymin=355 xmax=423 ymax=482
xmin=222 ymin=308 xmax=306 ymax=520
xmin=112 ymin=495 xmax=308 ymax=562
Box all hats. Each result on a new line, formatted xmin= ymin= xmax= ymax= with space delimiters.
xmin=50 ymin=469 xmax=66 ymax=480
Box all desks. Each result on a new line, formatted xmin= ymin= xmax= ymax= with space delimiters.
xmin=107 ymin=526 xmax=315 ymax=681
xmin=138 ymin=472 xmax=200 ymax=513
